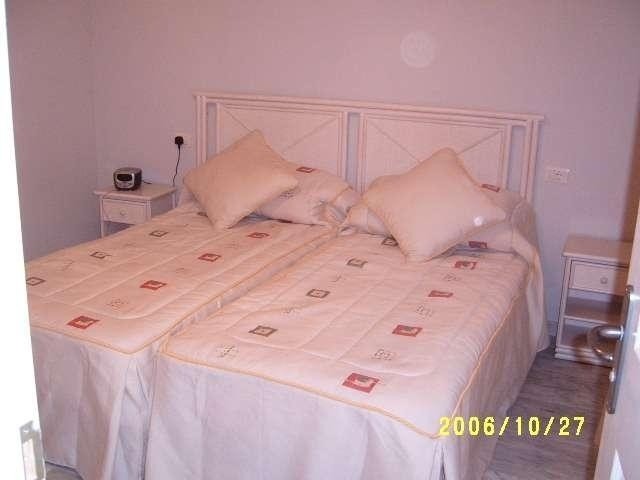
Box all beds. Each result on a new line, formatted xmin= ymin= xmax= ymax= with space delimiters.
xmin=25 ymin=91 xmax=353 ymax=480
xmin=141 ymin=102 xmax=550 ymax=480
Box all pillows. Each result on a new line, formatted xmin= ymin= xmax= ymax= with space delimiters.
xmin=342 ymin=172 xmax=534 ymax=262
xmin=364 ymin=147 xmax=508 ymax=264
xmin=178 ymin=164 xmax=355 ymax=227
xmin=183 ymin=129 xmax=297 ymax=231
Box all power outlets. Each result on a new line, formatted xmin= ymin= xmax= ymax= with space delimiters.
xmin=170 ymin=134 xmax=184 ymax=143
xmin=543 ymin=166 xmax=569 ymax=184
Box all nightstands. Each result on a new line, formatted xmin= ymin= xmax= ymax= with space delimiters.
xmin=555 ymin=236 xmax=630 ymax=369
xmin=91 ymin=186 xmax=175 ymax=238
